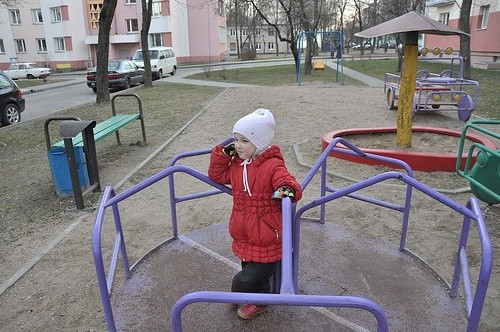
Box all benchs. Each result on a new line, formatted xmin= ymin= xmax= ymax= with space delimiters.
xmin=56 ymin=64 xmax=73 ymax=73
xmin=44 ymin=93 xmax=147 ymax=191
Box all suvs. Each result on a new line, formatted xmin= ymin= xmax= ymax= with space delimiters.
xmin=0 ymin=70 xmax=25 ymax=127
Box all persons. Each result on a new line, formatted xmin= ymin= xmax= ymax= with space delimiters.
xmin=206 ymin=108 xmax=302 ymax=320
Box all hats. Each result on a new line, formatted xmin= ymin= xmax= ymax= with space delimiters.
xmin=233 ymin=108 xmax=276 ymax=150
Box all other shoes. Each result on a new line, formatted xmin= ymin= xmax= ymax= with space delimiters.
xmin=227 ymin=303 xmax=237 ymax=308
xmin=237 ymin=304 xmax=268 ymax=319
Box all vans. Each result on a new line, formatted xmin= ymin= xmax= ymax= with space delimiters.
xmin=130 ymin=46 xmax=177 ymax=79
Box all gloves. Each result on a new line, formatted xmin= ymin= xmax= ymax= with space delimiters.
xmin=221 ymin=142 xmax=236 ymax=155
xmin=271 ymin=186 xmax=295 ymax=203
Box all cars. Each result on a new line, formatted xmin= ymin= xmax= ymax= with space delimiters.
xmin=86 ymin=59 xmax=145 ymax=93
xmin=353 ymin=42 xmax=374 ymax=50
xmin=397 ymin=43 xmax=422 ymax=56
xmin=3 ymin=63 xmax=52 ymax=80
